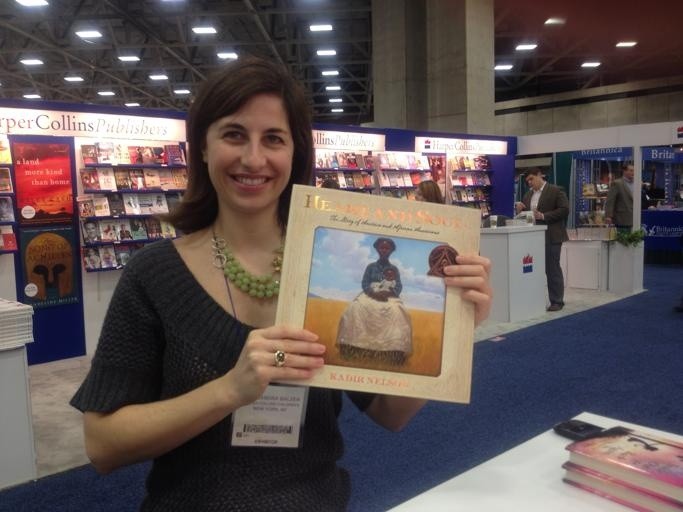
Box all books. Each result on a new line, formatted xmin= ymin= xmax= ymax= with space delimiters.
xmin=0 ymin=140 xmax=491 ymax=270
xmin=560 ymin=427 xmax=682 ymax=512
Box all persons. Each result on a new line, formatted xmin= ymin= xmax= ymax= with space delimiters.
xmin=373 ymin=268 xmax=396 ymax=293
xmin=335 ymin=238 xmax=416 ymax=365
xmin=605 ymin=162 xmax=634 ymax=231
xmin=69 ymin=58 xmax=496 ymax=510
xmin=514 ymin=168 xmax=571 ymax=311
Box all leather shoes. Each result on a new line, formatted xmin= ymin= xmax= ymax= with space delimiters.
xmin=548 ymin=303 xmax=563 ymax=311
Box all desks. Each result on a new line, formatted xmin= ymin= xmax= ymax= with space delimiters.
xmin=386 ymin=410 xmax=682 ymax=512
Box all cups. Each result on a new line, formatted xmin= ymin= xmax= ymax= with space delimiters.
xmin=489 ymin=214 xmax=498 ymax=228
xmin=526 ymin=213 xmax=535 ymax=228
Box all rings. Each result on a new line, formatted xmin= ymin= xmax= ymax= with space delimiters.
xmin=273 ymin=349 xmax=285 ymax=368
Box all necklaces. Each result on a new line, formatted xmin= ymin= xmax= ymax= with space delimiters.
xmin=212 ymin=223 xmax=285 ymax=297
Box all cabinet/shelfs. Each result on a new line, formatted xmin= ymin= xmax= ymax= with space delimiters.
xmin=77 ymin=161 xmax=191 ymax=277
xmin=0 ymin=163 xmax=20 ymax=254
xmin=309 ymin=165 xmax=493 ymax=221
